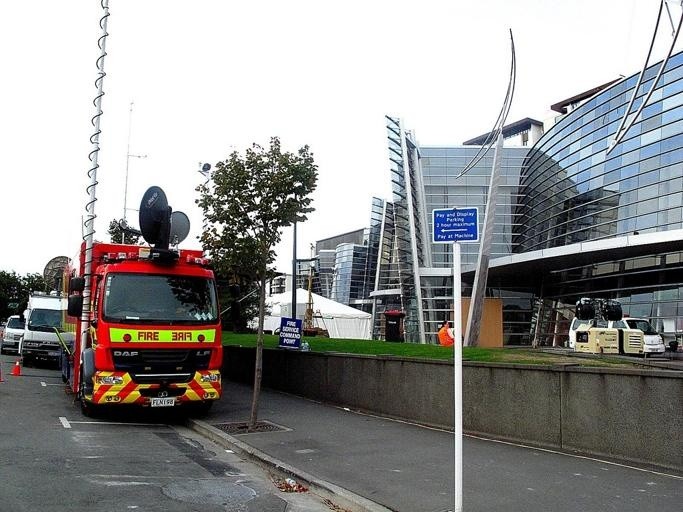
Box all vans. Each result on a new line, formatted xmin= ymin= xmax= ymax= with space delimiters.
xmin=567 ymin=316 xmax=666 ymax=357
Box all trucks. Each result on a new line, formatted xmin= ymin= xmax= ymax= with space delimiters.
xmin=18 ymin=288 xmax=70 ymax=372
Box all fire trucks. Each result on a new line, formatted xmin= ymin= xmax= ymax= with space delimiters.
xmin=59 ymin=238 xmax=226 ymax=417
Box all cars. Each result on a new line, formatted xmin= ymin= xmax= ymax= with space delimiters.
xmin=0 ymin=314 xmax=24 ymax=356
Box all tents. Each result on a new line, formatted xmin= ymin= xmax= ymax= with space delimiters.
xmin=246 ymin=286 xmax=372 ymax=339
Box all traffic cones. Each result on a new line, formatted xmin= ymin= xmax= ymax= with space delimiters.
xmin=10 ymin=358 xmax=21 ymax=379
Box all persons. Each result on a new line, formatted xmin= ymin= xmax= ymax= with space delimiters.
xmin=436 ymin=320 xmax=454 ymax=347
